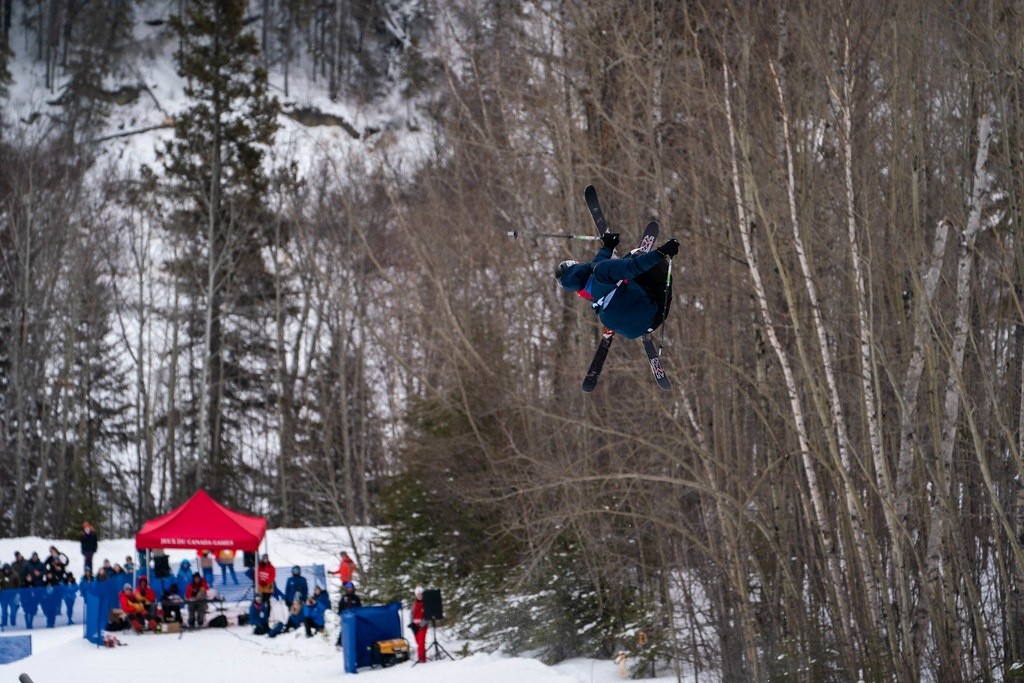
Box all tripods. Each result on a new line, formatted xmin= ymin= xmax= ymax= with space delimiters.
xmin=412 ymin=619 xmax=454 ymax=668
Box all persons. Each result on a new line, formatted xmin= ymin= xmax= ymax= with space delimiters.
xmin=556 ymin=228 xmax=680 ymax=337
xmin=0 ymin=520 xmax=427 ymax=663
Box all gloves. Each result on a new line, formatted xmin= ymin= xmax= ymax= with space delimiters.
xmin=602 ymin=232 xmax=620 ymax=247
xmin=659 ymin=238 xmax=679 ymax=258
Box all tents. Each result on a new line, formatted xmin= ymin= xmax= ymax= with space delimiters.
xmin=135 ymin=488 xmax=268 ymax=598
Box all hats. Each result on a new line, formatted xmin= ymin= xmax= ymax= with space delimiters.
xmin=193 ymin=572 xmax=201 ymax=578
xmin=254 ymin=592 xmax=262 ymax=597
xmin=264 ymin=554 xmax=268 ymax=558
xmin=555 ymin=260 xmax=578 ymax=286
xmin=415 ymin=587 xmax=424 ymax=595
xmin=124 ymin=582 xmax=132 ymax=590
xmin=82 ymin=521 xmax=91 ymax=528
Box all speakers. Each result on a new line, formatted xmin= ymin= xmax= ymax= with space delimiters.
xmin=243 ymin=549 xmax=255 ymax=567
xmin=423 ymin=589 xmax=443 ymax=621
xmin=154 ymin=554 xmax=170 ymax=579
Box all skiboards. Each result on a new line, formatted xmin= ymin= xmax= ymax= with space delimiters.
xmin=581 ymin=184 xmax=673 ymax=393
